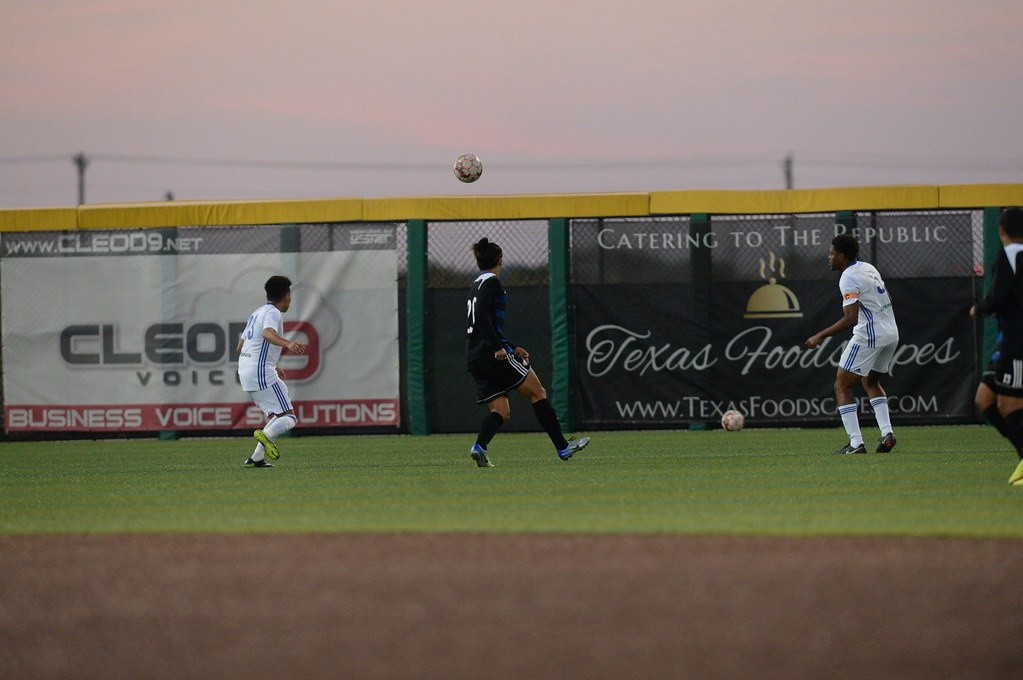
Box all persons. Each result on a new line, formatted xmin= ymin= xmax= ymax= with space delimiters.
xmin=464 ymin=238 xmax=591 ymax=467
xmin=236 ymin=276 xmax=305 ymax=468
xmin=969 ymin=207 xmax=1023 ymax=486
xmin=805 ymin=235 xmax=900 ymax=453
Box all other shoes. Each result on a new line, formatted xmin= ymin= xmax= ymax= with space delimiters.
xmin=1008 ymin=458 xmax=1023 ymax=486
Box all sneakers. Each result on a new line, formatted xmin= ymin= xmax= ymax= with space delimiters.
xmin=833 ymin=442 xmax=867 ymax=455
xmin=470 ymin=444 xmax=494 ymax=467
xmin=876 ymin=432 xmax=897 ymax=453
xmin=254 ymin=429 xmax=280 ymax=460
xmin=557 ymin=436 xmax=590 ymax=460
xmin=244 ymin=458 xmax=275 ymax=467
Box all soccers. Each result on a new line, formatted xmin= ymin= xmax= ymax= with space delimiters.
xmin=453 ymin=153 xmax=483 ymax=184
xmin=721 ymin=409 xmax=745 ymax=431
